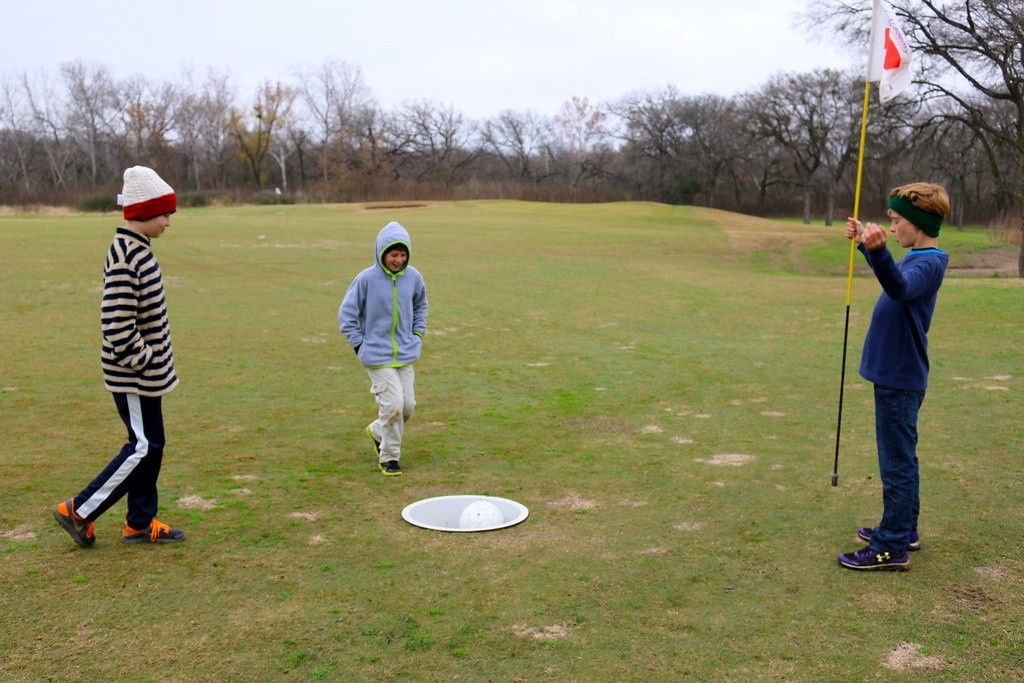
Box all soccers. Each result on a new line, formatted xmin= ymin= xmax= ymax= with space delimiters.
xmin=459 ymin=501 xmax=504 ymax=533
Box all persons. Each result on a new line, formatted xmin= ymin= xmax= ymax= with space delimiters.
xmin=837 ymin=183 xmax=951 ymax=571
xmin=52 ymin=164 xmax=185 ymax=547
xmin=338 ymin=222 xmax=428 ymax=476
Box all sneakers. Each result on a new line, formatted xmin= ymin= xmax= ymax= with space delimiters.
xmin=51 ymin=498 xmax=96 ymax=549
xmin=366 ymin=424 xmax=381 ymax=457
xmin=378 ymin=460 xmax=402 ymax=476
xmin=837 ymin=545 xmax=911 ymax=573
xmin=857 ymin=526 xmax=921 ymax=552
xmin=121 ymin=517 xmax=184 ymax=543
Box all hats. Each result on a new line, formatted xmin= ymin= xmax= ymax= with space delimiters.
xmin=117 ymin=165 xmax=177 ymax=221
